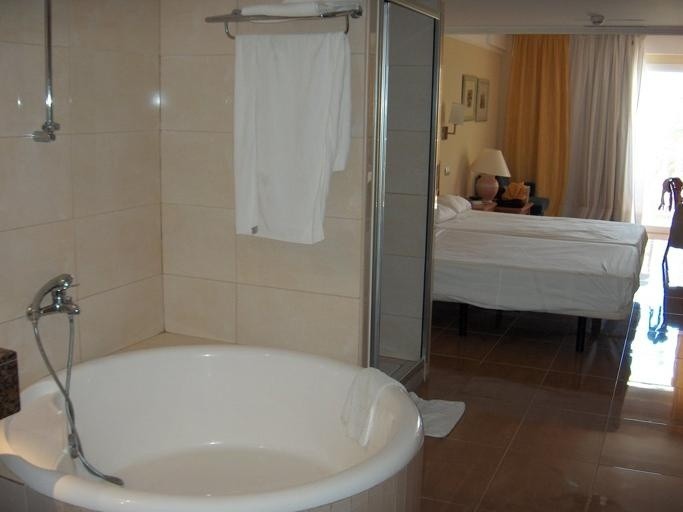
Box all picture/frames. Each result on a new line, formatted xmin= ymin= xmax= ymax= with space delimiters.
xmin=461 ymin=72 xmax=490 ymax=123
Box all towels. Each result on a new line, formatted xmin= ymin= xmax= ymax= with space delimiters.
xmin=232 ymin=1 xmax=352 ymax=244
xmin=338 ymin=367 xmax=465 ymax=447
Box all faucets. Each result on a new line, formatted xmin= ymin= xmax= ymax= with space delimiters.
xmin=57 ymin=302 xmax=81 ymax=315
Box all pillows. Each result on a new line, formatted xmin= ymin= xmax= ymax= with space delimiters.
xmin=434 ymin=194 xmax=472 ymax=223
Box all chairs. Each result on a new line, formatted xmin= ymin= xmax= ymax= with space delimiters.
xmin=658 ymin=177 xmax=682 ymax=270
xmin=654 ymin=261 xmax=683 ymax=335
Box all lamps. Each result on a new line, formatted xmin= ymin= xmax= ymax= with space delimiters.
xmin=469 ymin=148 xmax=511 ymax=201
xmin=439 ymin=103 xmax=465 ymax=141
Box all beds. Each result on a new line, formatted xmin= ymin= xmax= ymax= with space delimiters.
xmin=434 ymin=192 xmax=647 ymax=352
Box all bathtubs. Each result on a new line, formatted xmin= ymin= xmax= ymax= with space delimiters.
xmin=0 ymin=345 xmax=425 ymax=512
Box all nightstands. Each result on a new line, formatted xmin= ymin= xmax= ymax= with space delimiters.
xmin=496 ymin=199 xmax=534 ymax=214
